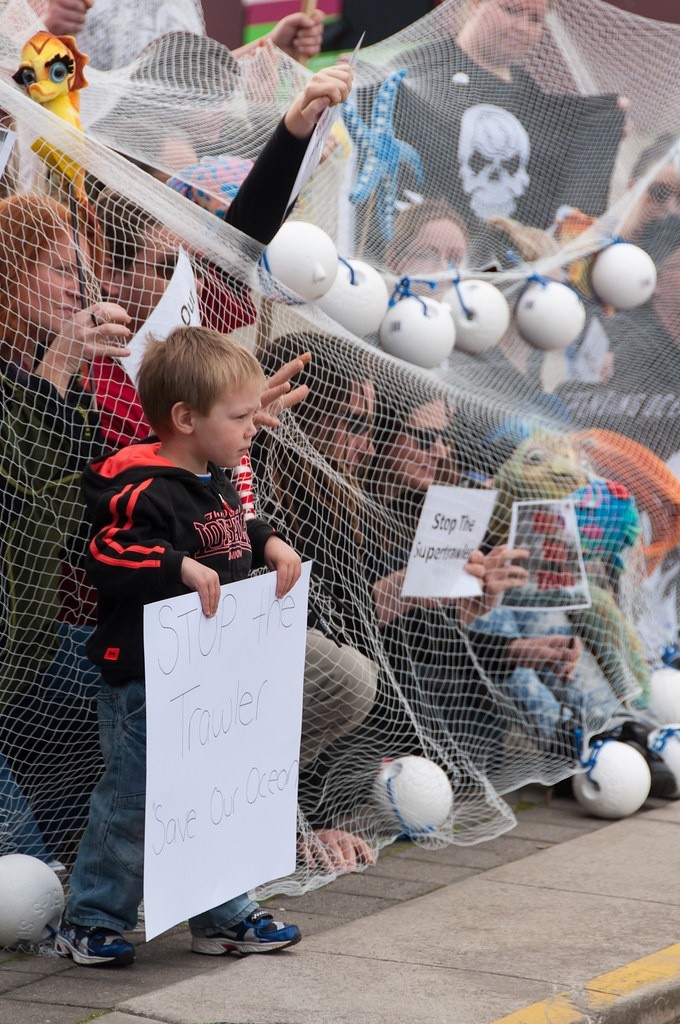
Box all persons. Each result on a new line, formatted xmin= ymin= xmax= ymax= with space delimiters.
xmin=55 ymin=322 xmax=306 ymax=969
xmin=0 ymin=199 xmax=132 ymax=846
xmin=255 ymin=335 xmax=582 ymax=870
xmin=0 ymin=0 xmax=678 ymax=467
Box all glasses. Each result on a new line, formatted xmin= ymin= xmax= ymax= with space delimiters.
xmin=396 ymin=423 xmax=453 ymax=450
xmin=333 ymin=405 xmax=375 ymax=434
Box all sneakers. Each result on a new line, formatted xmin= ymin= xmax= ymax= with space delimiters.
xmin=191 ymin=906 xmax=302 ymax=954
xmin=54 ymin=917 xmax=136 ymax=969
